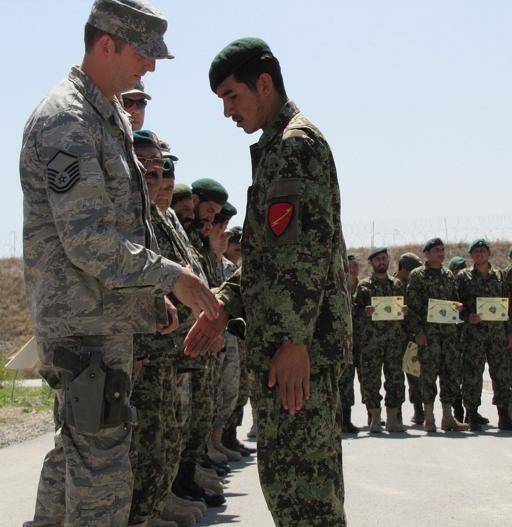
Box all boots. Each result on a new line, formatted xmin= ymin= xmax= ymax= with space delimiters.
xmin=340 ymin=402 xmax=512 ymax=433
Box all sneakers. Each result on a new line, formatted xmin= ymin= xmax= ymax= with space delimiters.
xmin=129 ymin=419 xmax=257 ymax=527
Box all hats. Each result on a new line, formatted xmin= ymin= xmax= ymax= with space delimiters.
xmin=208 ymin=37 xmax=272 ymax=93
xmin=367 ymin=238 xmax=490 ymax=272
xmin=85 ymin=0 xmax=244 ymax=245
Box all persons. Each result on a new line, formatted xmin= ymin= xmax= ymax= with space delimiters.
xmin=354 ymin=247 xmax=408 ymax=433
xmin=392 ymin=252 xmax=424 ymax=423
xmin=455 ymin=239 xmax=512 ymax=432
xmin=183 ymin=39 xmax=354 ymax=527
xmin=347 ymin=252 xmax=386 ymax=426
xmin=339 ymin=362 xmax=360 ymax=434
xmin=405 ymin=238 xmax=470 ymax=431
xmin=19 ymin=1 xmax=218 ymax=527
xmin=121 ymin=74 xmax=259 ymax=527
xmin=447 ymin=255 xmax=490 ymax=424
xmin=500 ymin=247 xmax=512 ymax=316
